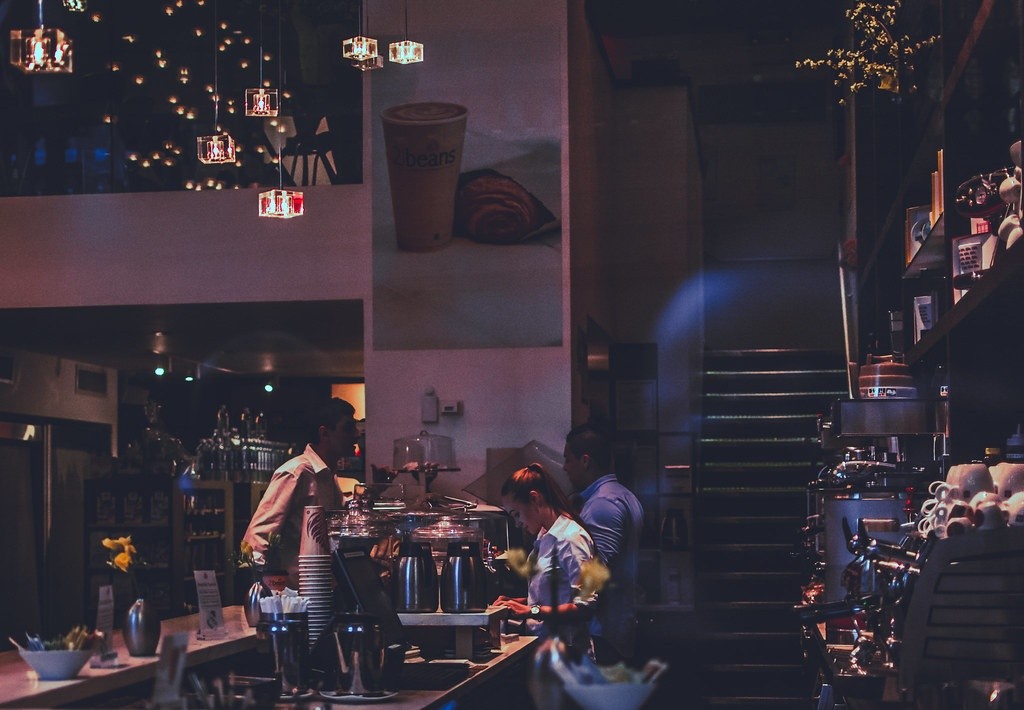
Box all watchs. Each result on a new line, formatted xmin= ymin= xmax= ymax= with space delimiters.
xmin=530 ymin=604 xmax=541 ymax=620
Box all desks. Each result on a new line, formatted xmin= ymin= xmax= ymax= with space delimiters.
xmin=273 ymin=636 xmax=541 ymax=710
xmin=0 ymin=605 xmax=271 ymax=710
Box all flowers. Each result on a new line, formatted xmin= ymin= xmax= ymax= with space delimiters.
xmin=102 ymin=536 xmax=144 ymax=598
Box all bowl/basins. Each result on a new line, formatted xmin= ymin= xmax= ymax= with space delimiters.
xmin=16 ymin=640 xmax=93 ymax=680
xmin=562 ymin=664 xmax=657 ymax=710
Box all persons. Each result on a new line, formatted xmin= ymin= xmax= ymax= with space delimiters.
xmin=563 ymin=426 xmax=643 ymax=662
xmin=244 ymin=397 xmax=358 ymax=591
xmin=493 ymin=463 xmax=606 ymax=710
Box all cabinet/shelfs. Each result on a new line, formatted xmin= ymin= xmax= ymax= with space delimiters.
xmin=86 ymin=469 xmax=172 ymax=628
xmin=861 ymin=0 xmax=1024 ymax=369
xmin=175 ymin=475 xmax=237 ymax=616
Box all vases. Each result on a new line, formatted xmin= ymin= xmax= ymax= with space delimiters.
xmin=121 ymin=598 xmax=161 ymax=656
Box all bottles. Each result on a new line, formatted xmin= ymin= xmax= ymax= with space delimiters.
xmin=981 ymin=422 xmax=1024 ymax=467
xmin=253 ymin=611 xmax=315 ymax=700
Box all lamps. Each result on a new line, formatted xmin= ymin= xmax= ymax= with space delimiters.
xmin=348 ymin=0 xmax=384 ymax=71
xmin=388 ymin=0 xmax=423 ymax=64
xmin=197 ymin=0 xmax=237 ymax=165
xmin=9 ymin=0 xmax=73 ymax=76
xmin=264 ymin=379 xmax=275 ymax=394
xmin=184 ymin=368 xmax=193 ymax=381
xmin=244 ymin=8 xmax=279 ymax=116
xmin=341 ymin=0 xmax=378 ymax=61
xmin=154 ymin=361 xmax=165 ymax=376
xmin=258 ymin=45 xmax=304 ymax=218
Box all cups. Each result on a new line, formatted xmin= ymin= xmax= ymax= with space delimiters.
xmin=918 ymin=302 xmax=932 ymax=329
xmin=957 ymin=242 xmax=981 ymax=274
xmin=297 ymin=504 xmax=333 ymax=654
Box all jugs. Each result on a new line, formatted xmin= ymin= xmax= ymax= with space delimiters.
xmin=393 ymin=540 xmax=439 ymax=614
xmin=307 ymin=605 xmax=405 ymax=702
xmin=437 ymin=543 xmax=489 ymax=612
